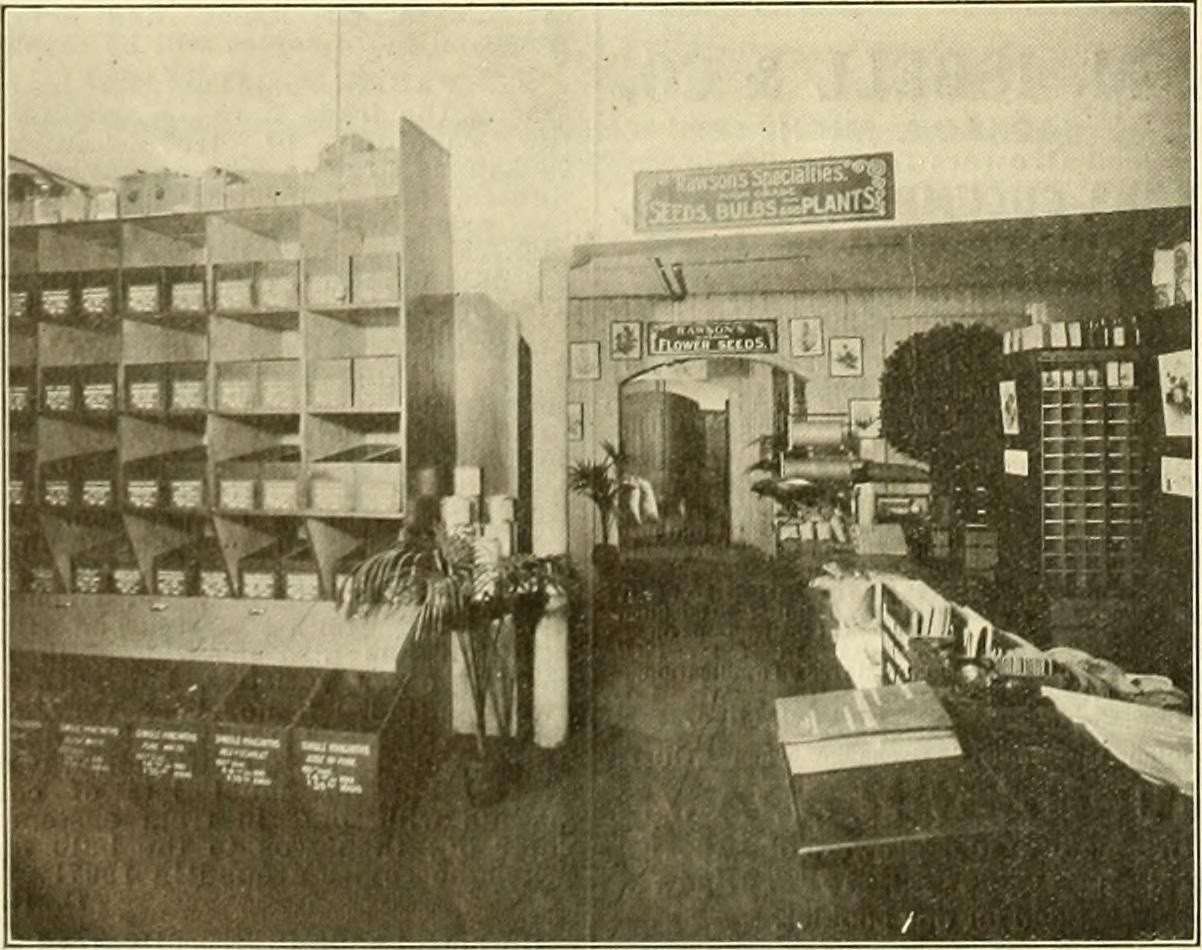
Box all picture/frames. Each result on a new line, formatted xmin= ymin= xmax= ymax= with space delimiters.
xmin=609 ymin=320 xmax=642 ymax=362
xmin=569 ymin=340 xmax=602 ymax=381
xmin=828 ymin=336 xmax=864 ymax=379
xmin=848 ymin=398 xmax=883 ymax=439
xmin=567 ymin=402 xmax=583 ymax=442
xmin=788 ymin=316 xmax=825 ymax=358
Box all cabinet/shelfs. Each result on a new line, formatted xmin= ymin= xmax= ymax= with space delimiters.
xmin=4 ymin=114 xmax=1195 ymax=942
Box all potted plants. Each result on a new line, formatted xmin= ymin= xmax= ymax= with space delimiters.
xmin=565 ymin=459 xmax=629 ymax=637
xmin=334 ymin=518 xmax=579 ymax=807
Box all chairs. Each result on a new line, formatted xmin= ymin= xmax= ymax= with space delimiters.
xmin=593 ymin=544 xmax=676 ymax=640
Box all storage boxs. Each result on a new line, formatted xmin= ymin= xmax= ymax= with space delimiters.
xmin=214 ymin=358 xmax=289 ymax=409
xmin=280 ymin=546 xmax=325 ymax=601
xmin=257 ymin=359 xmax=303 ymax=410
xmin=160 ymin=452 xmax=208 ymax=507
xmin=123 ymin=445 xmax=207 ymax=509
xmin=166 ymin=361 xmax=208 ymax=408
xmin=353 ymin=448 xmax=400 ymax=513
xmin=152 ymin=538 xmax=217 ymax=597
xmin=75 ymin=454 xmax=115 ymax=507
xmin=237 ymin=539 xmax=310 ymax=598
xmin=353 ymin=356 xmax=401 ymax=409
xmin=309 ymin=444 xmax=399 ymax=514
xmin=192 ymin=548 xmax=234 ymax=598
xmin=332 ymin=543 xmax=384 ymax=603
xmin=107 ymin=548 xmax=144 ymax=595
xmin=70 ymin=534 xmax=129 ymax=596
xmin=39 ymin=449 xmax=117 ymax=505
xmin=214 ymin=445 xmax=297 ymax=510
xmin=258 ymin=451 xmax=297 ymax=511
xmin=308 ymin=354 xmax=376 ymax=408
